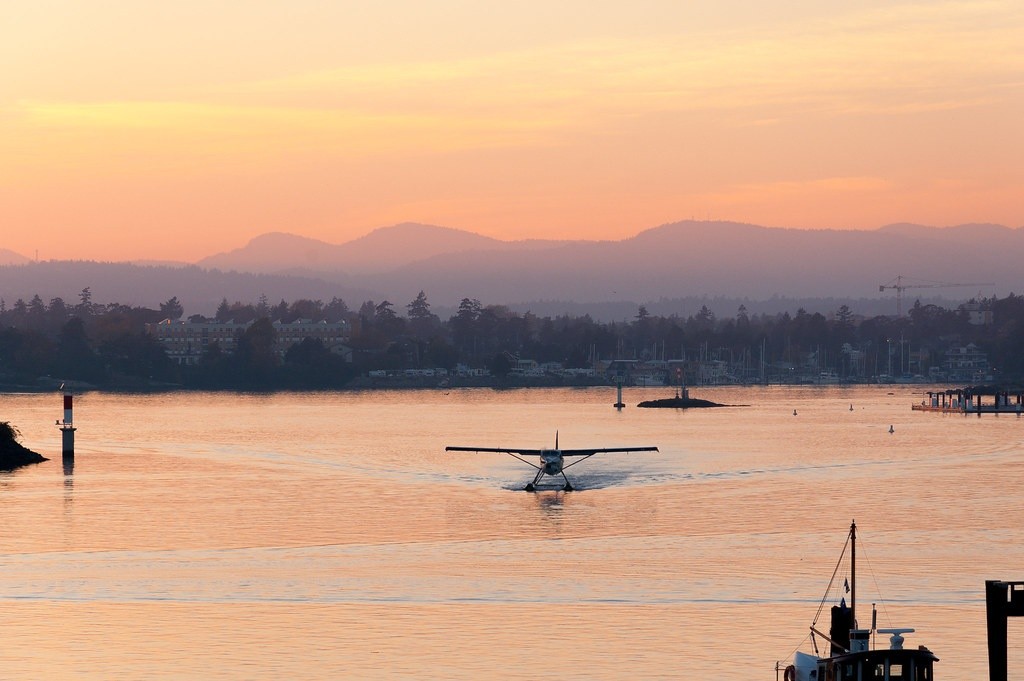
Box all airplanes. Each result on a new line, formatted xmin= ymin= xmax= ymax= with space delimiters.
xmin=446 ymin=431 xmax=661 ymax=494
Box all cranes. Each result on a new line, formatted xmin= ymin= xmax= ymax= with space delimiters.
xmin=878 ymin=276 xmax=998 ymax=319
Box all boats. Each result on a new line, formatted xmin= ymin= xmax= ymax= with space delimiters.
xmin=777 ymin=521 xmax=942 ymax=681
